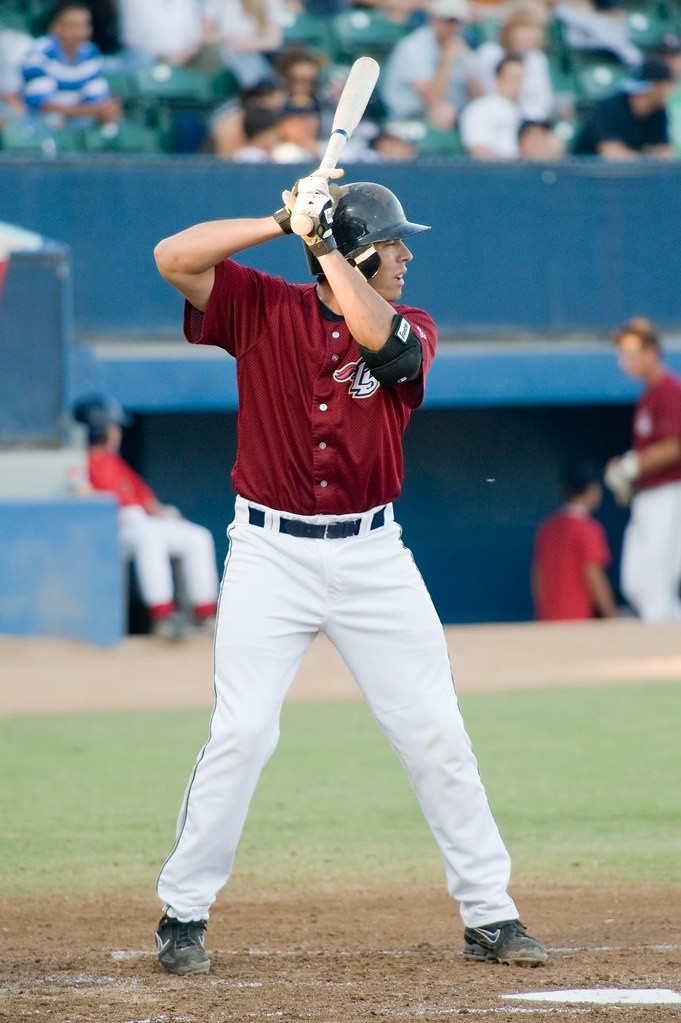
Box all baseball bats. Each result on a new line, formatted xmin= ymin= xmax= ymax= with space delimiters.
xmin=288 ymin=54 xmax=381 ymax=236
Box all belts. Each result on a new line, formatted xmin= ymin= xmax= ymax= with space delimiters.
xmin=249 ymin=506 xmax=385 ymax=538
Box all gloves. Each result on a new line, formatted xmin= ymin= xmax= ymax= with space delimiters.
xmin=274 ymin=167 xmax=348 ymax=258
xmin=605 ymin=452 xmax=639 ymax=506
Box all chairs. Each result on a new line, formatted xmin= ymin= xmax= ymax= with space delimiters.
xmin=0 ymin=0 xmax=680 ymax=160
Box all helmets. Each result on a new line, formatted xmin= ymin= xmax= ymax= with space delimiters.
xmin=302 ymin=182 xmax=431 ymax=281
xmin=74 ymin=396 xmax=131 ymax=444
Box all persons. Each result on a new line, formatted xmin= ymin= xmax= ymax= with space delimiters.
xmin=0 ymin=0 xmax=681 ymax=164
xmin=154 ymin=169 xmax=549 ymax=976
xmin=534 ymin=316 xmax=681 ymax=627
xmin=66 ymin=391 xmax=221 ymax=641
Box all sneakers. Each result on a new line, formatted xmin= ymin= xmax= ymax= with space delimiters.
xmin=156 ymin=907 xmax=210 ymax=976
xmin=464 ymin=919 xmax=548 ymax=968
